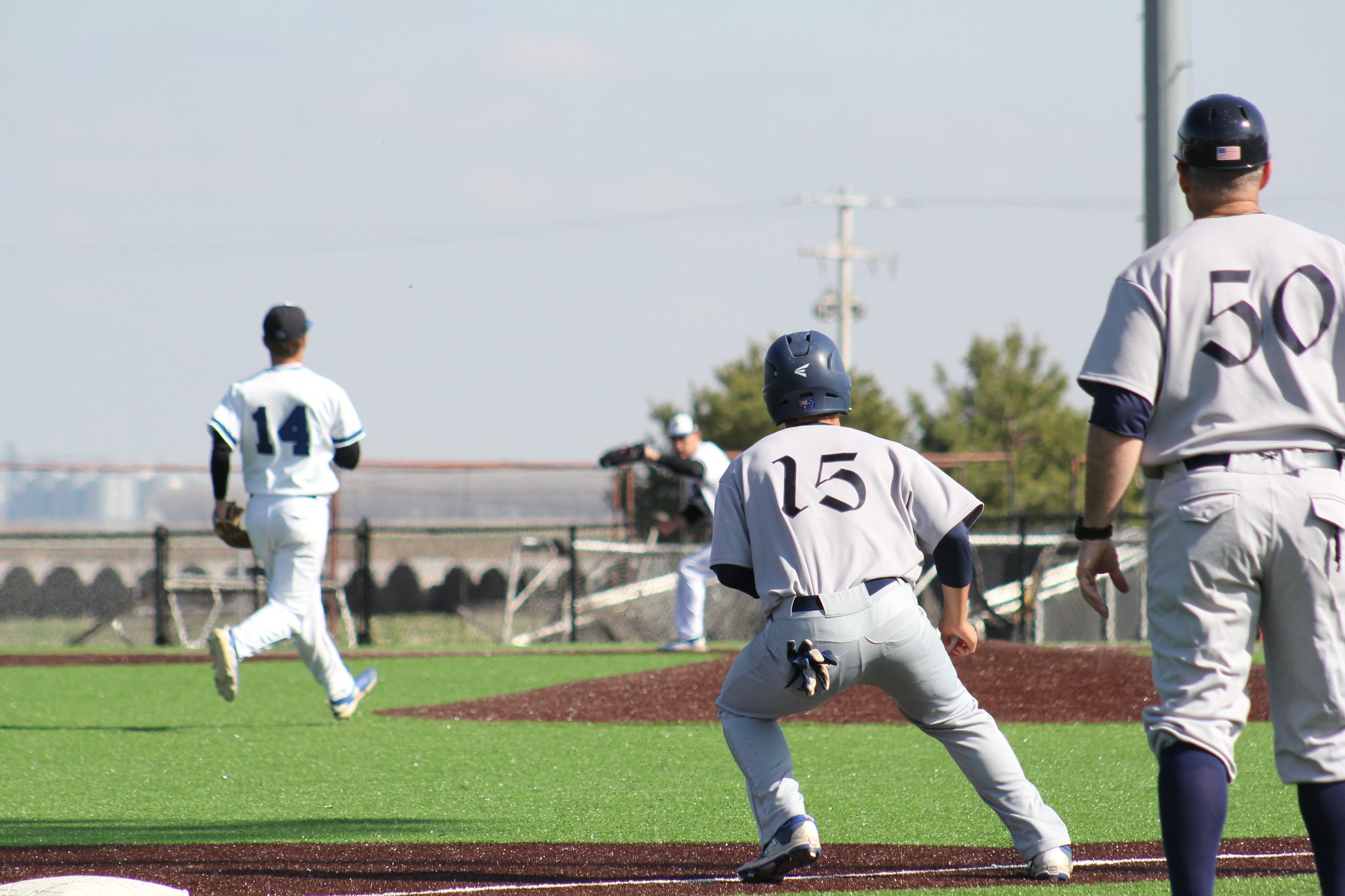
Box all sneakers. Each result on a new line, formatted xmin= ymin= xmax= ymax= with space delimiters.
xmin=331 ymin=669 xmax=377 ymax=717
xmin=737 ymin=815 xmax=821 ymax=881
xmin=209 ymin=625 xmax=239 ymax=699
xmin=1031 ymin=846 xmax=1072 ymax=879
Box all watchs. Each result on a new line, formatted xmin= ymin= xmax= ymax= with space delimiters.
xmin=1071 ymin=514 xmax=1114 ymax=539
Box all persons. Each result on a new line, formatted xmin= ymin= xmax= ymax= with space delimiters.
xmin=632 ymin=413 xmax=730 ymax=652
xmin=205 ymin=304 xmax=378 ymax=720
xmin=708 ymin=330 xmax=1076 ymax=879
xmin=1078 ymin=93 xmax=1345 ymax=896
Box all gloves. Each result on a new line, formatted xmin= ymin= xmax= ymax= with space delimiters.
xmin=784 ymin=640 xmax=837 ymax=696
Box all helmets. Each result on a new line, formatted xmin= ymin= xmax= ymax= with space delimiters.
xmin=1174 ymin=94 xmax=1268 ymax=168
xmin=762 ymin=331 xmax=851 ymax=426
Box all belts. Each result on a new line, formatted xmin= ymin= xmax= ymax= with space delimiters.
xmin=1144 ymin=450 xmax=1342 ymax=481
xmin=771 ymin=578 xmax=896 ymax=620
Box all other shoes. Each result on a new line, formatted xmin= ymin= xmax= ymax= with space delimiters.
xmin=657 ymin=636 xmax=707 ymax=651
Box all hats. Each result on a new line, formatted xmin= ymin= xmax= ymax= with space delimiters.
xmin=264 ymin=306 xmax=311 ymax=340
xmin=667 ymin=414 xmax=693 ymax=435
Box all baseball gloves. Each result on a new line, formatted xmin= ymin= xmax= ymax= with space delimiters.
xmin=211 ymin=505 xmax=253 ymax=550
